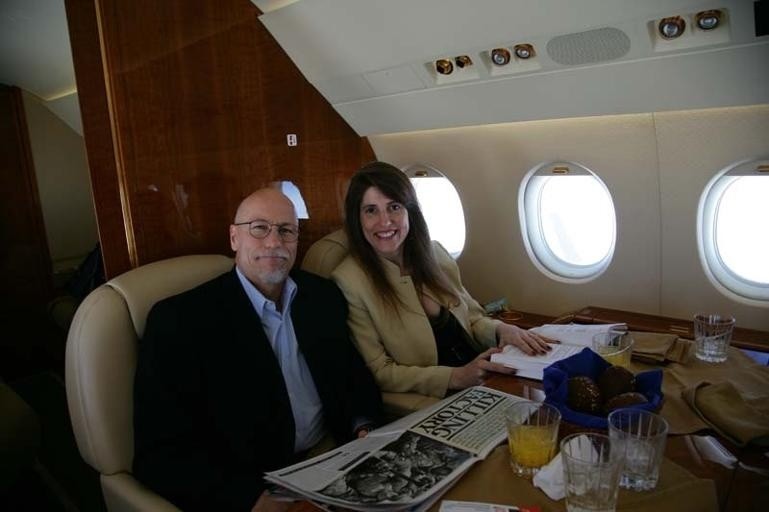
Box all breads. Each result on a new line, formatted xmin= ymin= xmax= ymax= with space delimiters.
xmin=567 ymin=365 xmax=648 ymax=418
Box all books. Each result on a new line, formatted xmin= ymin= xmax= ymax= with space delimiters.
xmin=488 ymin=319 xmax=630 ymax=381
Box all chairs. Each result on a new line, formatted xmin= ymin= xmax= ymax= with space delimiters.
xmin=65 ymin=225 xmax=441 ymax=510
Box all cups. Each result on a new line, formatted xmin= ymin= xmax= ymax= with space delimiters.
xmin=692 ymin=309 xmax=735 ymax=364
xmin=502 ymin=400 xmax=669 ymax=512
xmin=590 ymin=333 xmax=634 ymax=374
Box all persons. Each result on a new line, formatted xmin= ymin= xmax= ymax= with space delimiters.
xmin=321 ymin=430 xmax=476 ymax=504
xmin=327 ymin=160 xmax=563 ymax=400
xmin=131 ymin=187 xmax=383 ymax=510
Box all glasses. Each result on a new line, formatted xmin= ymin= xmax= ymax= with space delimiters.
xmin=235 ymin=218 xmax=300 ymax=241
